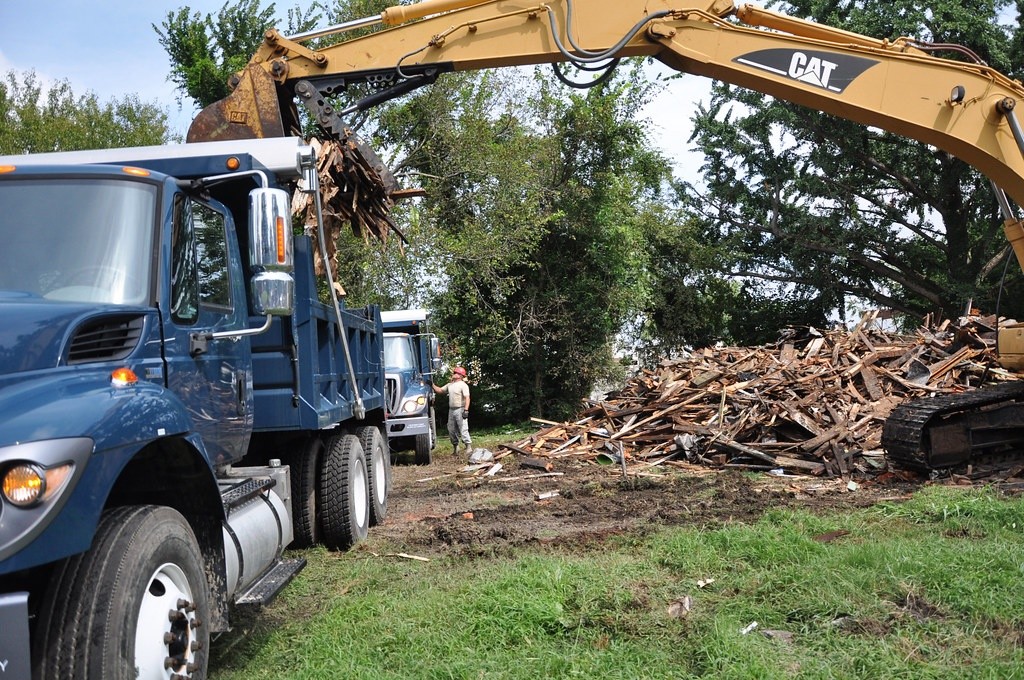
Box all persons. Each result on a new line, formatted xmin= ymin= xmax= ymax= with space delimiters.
xmin=426 ymin=367 xmax=473 ymax=455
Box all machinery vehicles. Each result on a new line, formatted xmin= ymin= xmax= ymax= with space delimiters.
xmin=185 ymin=0 xmax=1024 ymax=485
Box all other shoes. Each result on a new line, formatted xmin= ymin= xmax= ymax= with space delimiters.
xmin=452 ymin=443 xmax=461 ymax=455
xmin=466 ymin=443 xmax=472 ymax=453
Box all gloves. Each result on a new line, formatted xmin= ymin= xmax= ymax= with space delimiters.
xmin=425 ymin=378 xmax=433 ymax=386
xmin=462 ymin=410 xmax=469 ymax=419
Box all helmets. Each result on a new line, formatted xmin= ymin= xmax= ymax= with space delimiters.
xmin=454 ymin=367 xmax=467 ymax=377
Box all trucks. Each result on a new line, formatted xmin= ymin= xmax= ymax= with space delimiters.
xmin=374 ymin=307 xmax=445 ymax=462
xmin=0 ymin=131 xmax=389 ymax=679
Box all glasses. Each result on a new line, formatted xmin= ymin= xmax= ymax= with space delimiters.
xmin=453 ymin=372 xmax=460 ymax=375
